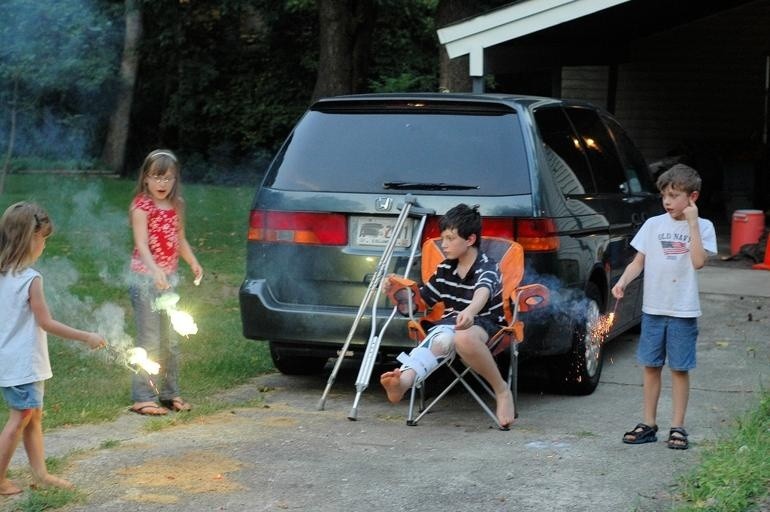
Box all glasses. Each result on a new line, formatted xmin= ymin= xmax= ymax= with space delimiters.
xmin=146 ymin=174 xmax=176 ymax=183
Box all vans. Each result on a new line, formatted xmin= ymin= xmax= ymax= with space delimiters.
xmin=240 ymin=92 xmax=667 ymax=396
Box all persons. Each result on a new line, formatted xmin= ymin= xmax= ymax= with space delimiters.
xmin=608 ymin=160 xmax=719 ymax=451
xmin=120 ymin=145 xmax=203 ymax=418
xmin=0 ymin=199 xmax=112 ymax=496
xmin=379 ymin=202 xmax=516 ymax=427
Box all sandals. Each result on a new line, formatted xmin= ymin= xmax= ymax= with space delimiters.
xmin=623 ymin=423 xmax=658 ymax=444
xmin=667 ymin=426 xmax=689 ymax=449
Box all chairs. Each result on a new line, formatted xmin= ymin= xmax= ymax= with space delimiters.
xmin=406 ymin=235 xmax=527 ymax=430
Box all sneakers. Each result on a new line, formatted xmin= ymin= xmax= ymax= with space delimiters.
xmin=0 ymin=477 xmax=66 ymax=496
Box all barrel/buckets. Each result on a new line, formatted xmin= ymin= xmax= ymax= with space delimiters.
xmin=729 ymin=208 xmax=766 ymax=258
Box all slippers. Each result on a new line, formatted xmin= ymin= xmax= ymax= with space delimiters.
xmin=129 ymin=402 xmax=169 ymax=416
xmin=161 ymin=397 xmax=192 ymax=412
